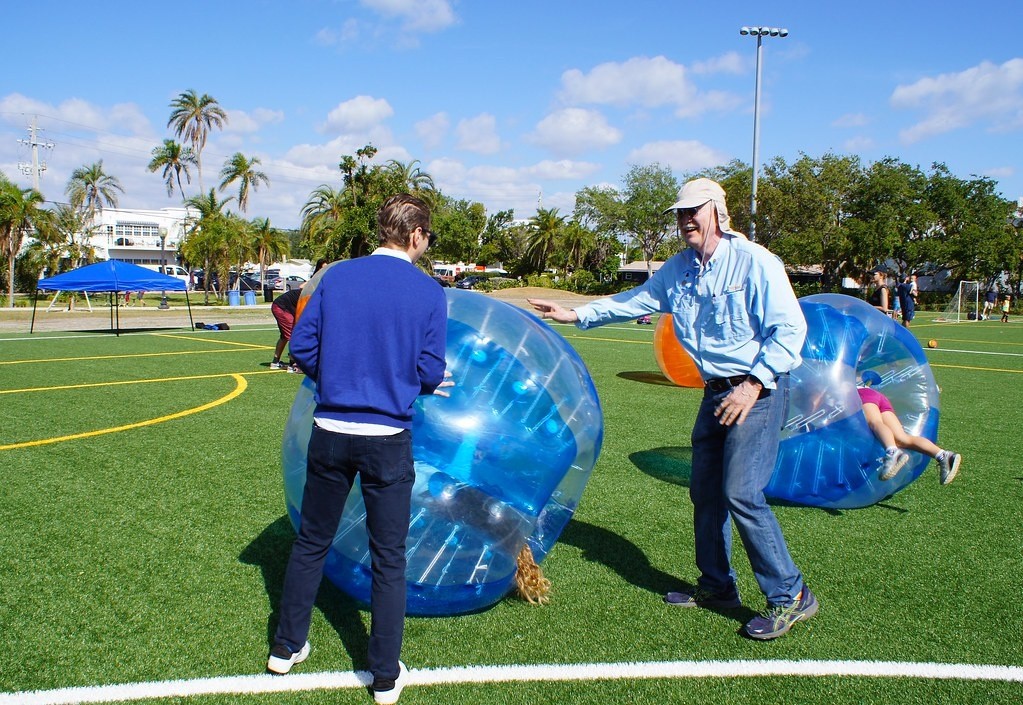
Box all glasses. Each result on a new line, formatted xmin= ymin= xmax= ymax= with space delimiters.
xmin=676 ymin=205 xmax=708 ymax=217
xmin=422 ymin=228 xmax=437 ymax=246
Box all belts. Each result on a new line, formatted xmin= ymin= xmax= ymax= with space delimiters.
xmin=705 ymin=375 xmax=749 ymax=391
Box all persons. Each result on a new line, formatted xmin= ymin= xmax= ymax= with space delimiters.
xmin=270 ymin=288 xmax=304 ymax=374
xmin=126 ymin=291 xmax=131 ymax=307
xmin=1001 ymin=295 xmax=1011 ymax=322
xmin=137 ymin=290 xmax=147 ymax=307
xmin=299 ymin=259 xmax=328 ymax=288
xmin=529 ymin=177 xmax=821 ymax=642
xmin=893 ymin=273 xmax=918 ymax=328
xmin=794 ymin=385 xmax=961 ymax=484
xmin=982 ymin=287 xmax=997 ymax=320
xmin=267 ymin=192 xmax=452 ymax=705
xmin=867 ymin=265 xmax=889 ymax=314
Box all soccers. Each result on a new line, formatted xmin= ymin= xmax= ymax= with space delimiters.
xmin=928 ymin=340 xmax=937 ymax=348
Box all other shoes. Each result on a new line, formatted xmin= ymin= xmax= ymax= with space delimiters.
xmin=1001 ymin=319 xmax=1004 ymax=323
xmin=1005 ymin=320 xmax=1008 ymax=323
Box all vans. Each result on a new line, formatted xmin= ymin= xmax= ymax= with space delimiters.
xmin=136 ymin=264 xmax=198 ymax=288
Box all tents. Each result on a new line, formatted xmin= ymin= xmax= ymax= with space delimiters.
xmin=30 ymin=259 xmax=194 ymax=336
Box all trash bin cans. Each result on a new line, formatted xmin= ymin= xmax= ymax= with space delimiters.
xmin=227 ymin=290 xmax=240 ymax=306
xmin=243 ymin=291 xmax=256 ymax=305
xmin=264 ymin=288 xmax=273 ymax=302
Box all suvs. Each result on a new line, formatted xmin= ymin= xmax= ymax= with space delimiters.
xmin=252 ymin=274 xmax=279 ymax=291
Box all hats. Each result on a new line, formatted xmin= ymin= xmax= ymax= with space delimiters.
xmin=867 ymin=265 xmax=886 ymax=274
xmin=662 ymin=177 xmax=745 ymax=239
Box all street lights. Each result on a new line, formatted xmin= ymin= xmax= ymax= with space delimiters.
xmin=158 ymin=226 xmax=169 ymax=309
xmin=739 ymin=25 xmax=788 ymax=241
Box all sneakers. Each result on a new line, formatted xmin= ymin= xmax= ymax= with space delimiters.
xmin=269 ymin=361 xmax=288 ymax=370
xmin=937 ymin=452 xmax=961 ymax=486
xmin=880 ymin=450 xmax=909 ymax=479
xmin=745 ymin=585 xmax=819 ymax=640
xmin=286 ymin=365 xmax=303 ymax=374
xmin=663 ymin=584 xmax=741 ymax=608
xmin=268 ymin=639 xmax=310 ymax=673
xmin=374 ymin=659 xmax=408 ymax=704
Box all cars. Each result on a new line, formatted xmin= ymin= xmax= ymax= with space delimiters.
xmin=197 ymin=272 xmax=261 ymax=294
xmin=267 ymin=275 xmax=306 ymax=290
xmin=456 ymin=275 xmax=490 ymax=290
xmin=432 ymin=277 xmax=451 ymax=288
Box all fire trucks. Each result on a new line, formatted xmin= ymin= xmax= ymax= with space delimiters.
xmin=433 ymin=264 xmax=486 ymax=284
